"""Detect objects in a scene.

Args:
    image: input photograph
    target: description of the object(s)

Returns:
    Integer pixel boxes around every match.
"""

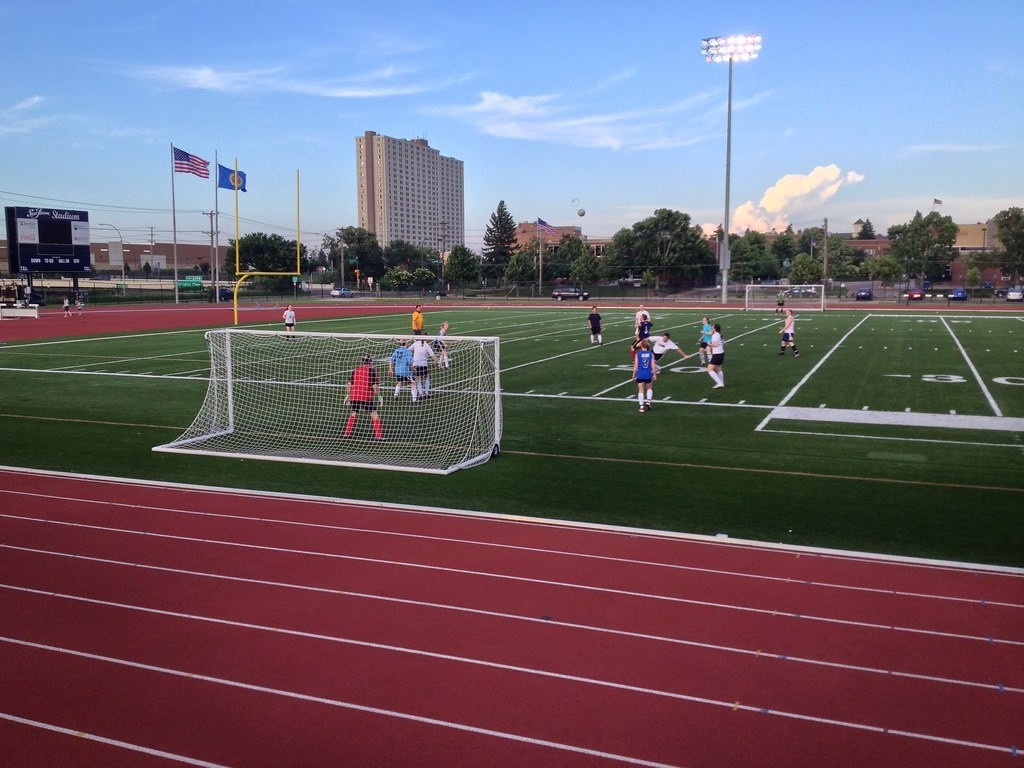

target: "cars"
[982,280,994,288]
[923,281,933,290]
[908,288,924,301]
[951,288,967,300]
[1007,289,1023,301]
[856,288,873,300]
[783,286,819,298]
[330,288,355,298]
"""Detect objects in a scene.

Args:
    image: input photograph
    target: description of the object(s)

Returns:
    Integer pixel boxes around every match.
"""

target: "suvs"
[210,288,233,301]
[552,288,590,301]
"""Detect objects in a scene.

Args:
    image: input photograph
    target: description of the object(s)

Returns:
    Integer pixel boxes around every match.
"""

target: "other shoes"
[424,393,435,399]
[341,437,353,442]
[411,399,419,404]
[438,365,445,370]
[418,394,426,399]
[443,366,453,370]
[639,406,646,413]
[375,437,387,443]
[393,396,399,401]
[774,313,783,316]
[712,384,725,388]
[794,353,800,358]
[700,362,708,366]
[646,401,651,410]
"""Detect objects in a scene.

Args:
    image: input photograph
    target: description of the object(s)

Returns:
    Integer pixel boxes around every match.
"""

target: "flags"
[537,218,557,236]
[812,240,817,247]
[174,146,210,179]
[218,164,246,193]
[935,199,942,204]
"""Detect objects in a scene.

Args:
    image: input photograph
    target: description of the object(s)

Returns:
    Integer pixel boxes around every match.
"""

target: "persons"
[282,304,297,343]
[389,338,420,404]
[63,295,72,317]
[411,305,424,342]
[776,289,785,313]
[707,323,726,388]
[628,304,689,375]
[408,331,438,400]
[375,279,380,298]
[778,309,800,358]
[588,306,603,345]
[631,340,657,413]
[75,299,82,315]
[698,316,713,366]
[343,354,383,440]
[434,320,451,369]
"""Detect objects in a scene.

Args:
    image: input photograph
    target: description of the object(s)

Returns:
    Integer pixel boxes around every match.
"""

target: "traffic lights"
[357,270,361,276]
[355,270,357,276]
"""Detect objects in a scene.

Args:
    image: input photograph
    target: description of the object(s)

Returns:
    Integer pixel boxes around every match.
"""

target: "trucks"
[995,284,1024,297]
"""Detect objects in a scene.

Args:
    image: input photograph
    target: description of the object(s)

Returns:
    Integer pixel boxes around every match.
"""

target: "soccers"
[578,208,585,217]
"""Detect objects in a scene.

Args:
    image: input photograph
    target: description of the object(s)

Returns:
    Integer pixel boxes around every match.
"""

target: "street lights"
[99,223,126,298]
[981,228,987,304]
[702,34,763,305]
[421,239,428,292]
